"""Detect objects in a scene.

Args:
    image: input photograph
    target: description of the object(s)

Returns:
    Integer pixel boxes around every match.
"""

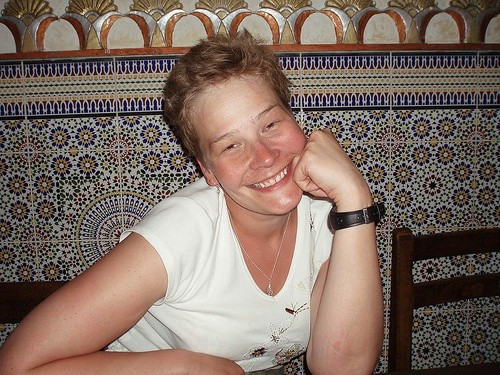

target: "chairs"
[386,226,500,375]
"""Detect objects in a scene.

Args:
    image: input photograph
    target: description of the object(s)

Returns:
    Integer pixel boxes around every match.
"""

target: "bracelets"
[329,201,387,230]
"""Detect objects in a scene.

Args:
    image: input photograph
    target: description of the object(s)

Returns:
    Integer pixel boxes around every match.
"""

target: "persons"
[0,29,385,375]
